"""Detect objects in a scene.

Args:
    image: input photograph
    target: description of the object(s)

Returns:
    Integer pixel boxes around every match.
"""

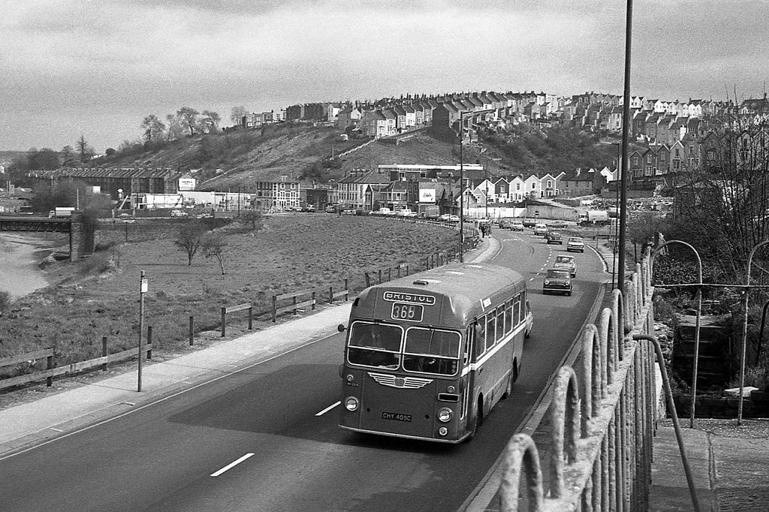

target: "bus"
[335,262,535,451]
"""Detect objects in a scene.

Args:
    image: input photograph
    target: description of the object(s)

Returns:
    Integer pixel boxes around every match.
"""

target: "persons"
[481,224,485,238]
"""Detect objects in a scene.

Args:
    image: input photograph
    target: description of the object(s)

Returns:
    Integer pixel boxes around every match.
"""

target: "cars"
[322,201,460,223]
[497,217,564,245]
[542,255,577,296]
[567,236,584,253]
